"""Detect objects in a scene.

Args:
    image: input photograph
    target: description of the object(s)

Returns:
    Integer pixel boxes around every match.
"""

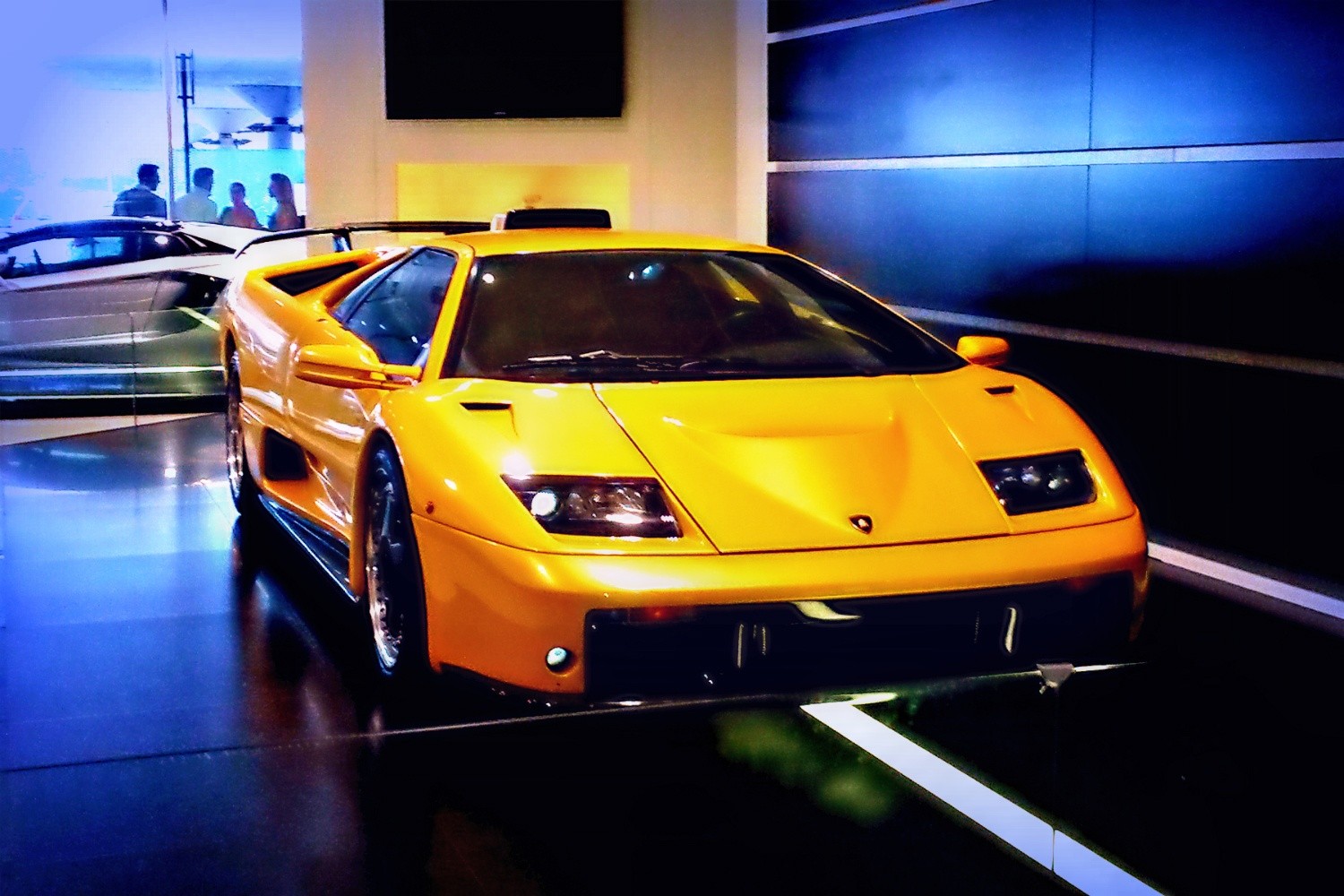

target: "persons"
[219,181,262,229]
[111,163,167,219]
[266,172,298,230]
[172,167,219,223]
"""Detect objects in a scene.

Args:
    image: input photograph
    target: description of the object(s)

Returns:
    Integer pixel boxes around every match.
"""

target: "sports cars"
[0,215,277,367]
[217,204,1151,706]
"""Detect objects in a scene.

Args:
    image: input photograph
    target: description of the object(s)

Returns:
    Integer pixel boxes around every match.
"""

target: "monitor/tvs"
[384,0,625,118]
